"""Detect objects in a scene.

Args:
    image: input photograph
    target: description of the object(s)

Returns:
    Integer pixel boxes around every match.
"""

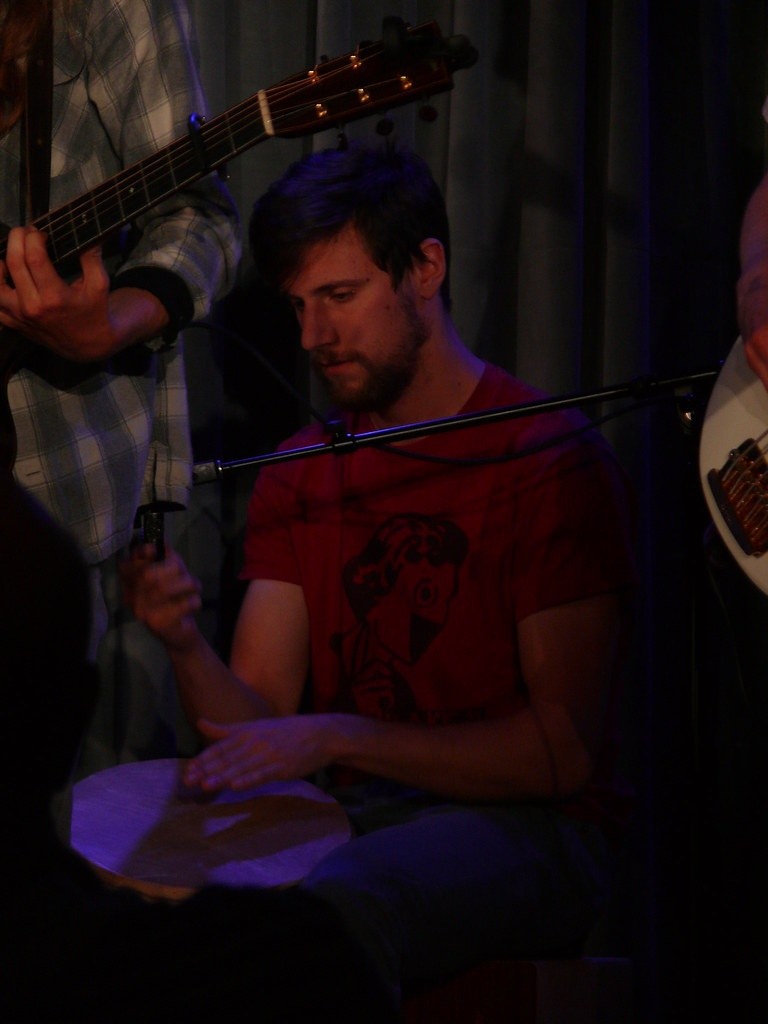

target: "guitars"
[0,17,479,305]
[700,334,768,596]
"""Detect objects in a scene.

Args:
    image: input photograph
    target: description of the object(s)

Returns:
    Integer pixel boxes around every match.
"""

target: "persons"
[68,149,619,1023]
[0,0,241,786]
[737,172,768,389]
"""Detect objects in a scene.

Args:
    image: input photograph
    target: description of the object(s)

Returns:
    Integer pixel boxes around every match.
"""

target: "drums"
[57,759,353,906]
